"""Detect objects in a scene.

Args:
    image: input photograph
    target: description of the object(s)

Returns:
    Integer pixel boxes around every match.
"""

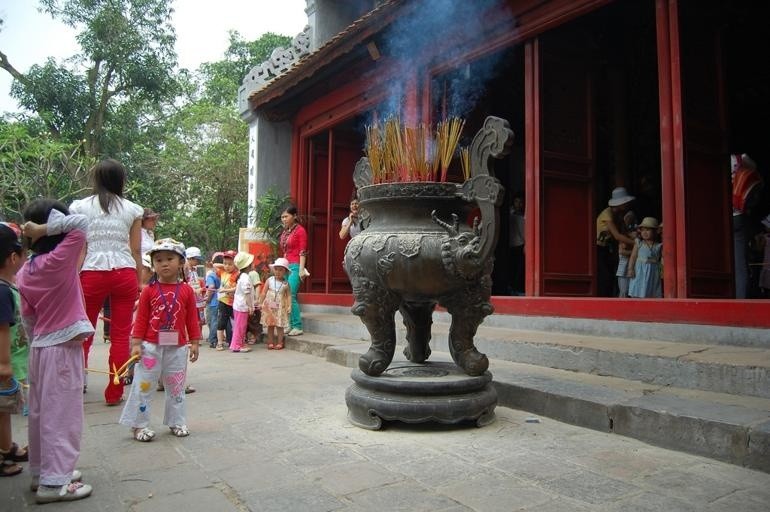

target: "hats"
[185,247,203,259]
[212,248,255,269]
[268,258,290,274]
[608,187,636,208]
[145,237,186,265]
[142,207,161,221]
[637,216,660,230]
[0,221,24,251]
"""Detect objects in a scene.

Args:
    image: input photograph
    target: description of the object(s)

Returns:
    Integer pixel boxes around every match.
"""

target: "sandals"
[0,441,29,477]
[266,342,285,350]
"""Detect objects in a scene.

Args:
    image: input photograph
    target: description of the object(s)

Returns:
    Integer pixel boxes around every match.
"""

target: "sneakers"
[156,383,196,394]
[124,375,134,385]
[30,469,93,504]
[210,338,256,352]
[284,326,304,336]
[133,428,156,442]
[168,423,190,438]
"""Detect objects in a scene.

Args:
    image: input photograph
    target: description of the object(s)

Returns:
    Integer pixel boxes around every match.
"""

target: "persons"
[492,194,525,296]
[731,153,770,299]
[14,198,92,503]
[68,159,145,406]
[597,187,663,298]
[339,193,361,239]
[0,222,31,476]
